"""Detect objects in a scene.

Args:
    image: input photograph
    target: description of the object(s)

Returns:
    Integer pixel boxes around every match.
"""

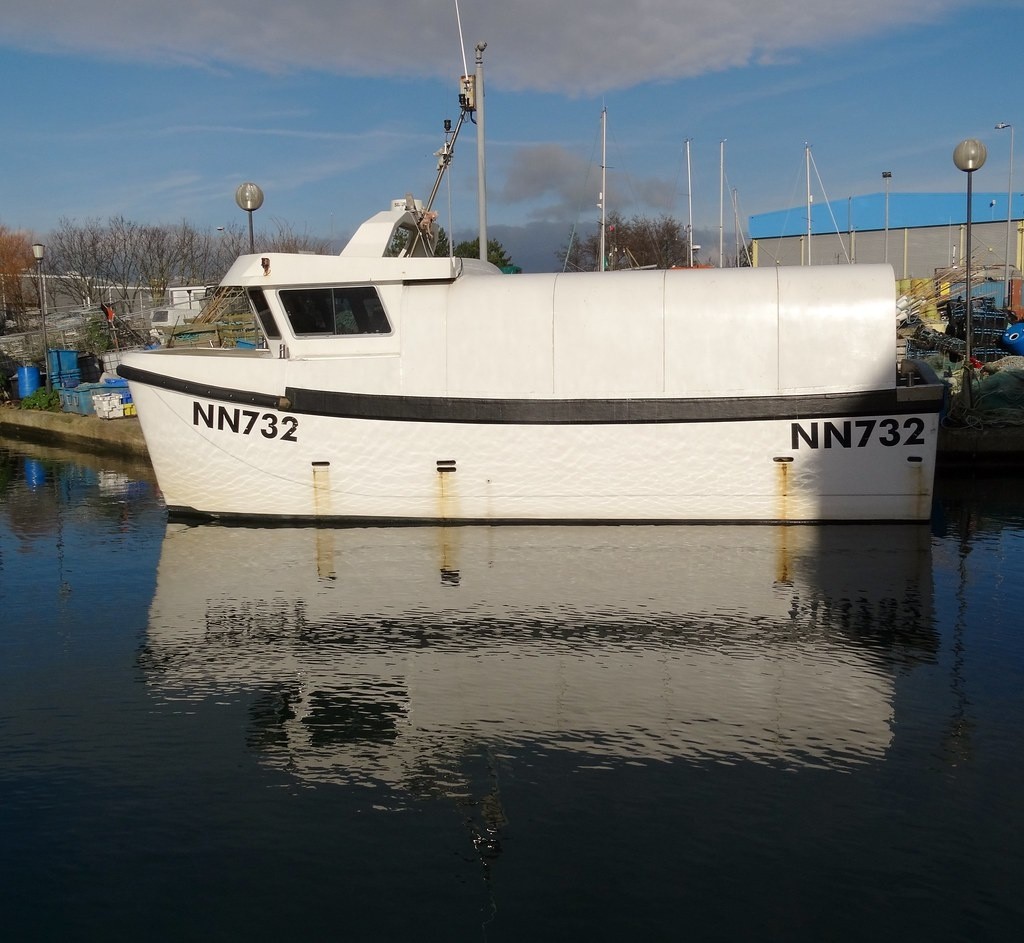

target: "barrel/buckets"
[1002,323,1024,357]
[18,366,41,399]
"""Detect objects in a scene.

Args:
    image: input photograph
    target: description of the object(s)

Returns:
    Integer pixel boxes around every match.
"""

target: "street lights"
[234,181,264,255]
[881,171,893,264]
[31,242,52,395]
[992,122,1015,309]
[951,138,988,411]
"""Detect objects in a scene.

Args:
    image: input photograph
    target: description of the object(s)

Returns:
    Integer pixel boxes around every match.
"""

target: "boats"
[115,1,946,526]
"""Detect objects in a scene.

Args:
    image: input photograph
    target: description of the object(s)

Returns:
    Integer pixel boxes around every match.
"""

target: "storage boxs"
[235,338,257,348]
[48,348,137,419]
